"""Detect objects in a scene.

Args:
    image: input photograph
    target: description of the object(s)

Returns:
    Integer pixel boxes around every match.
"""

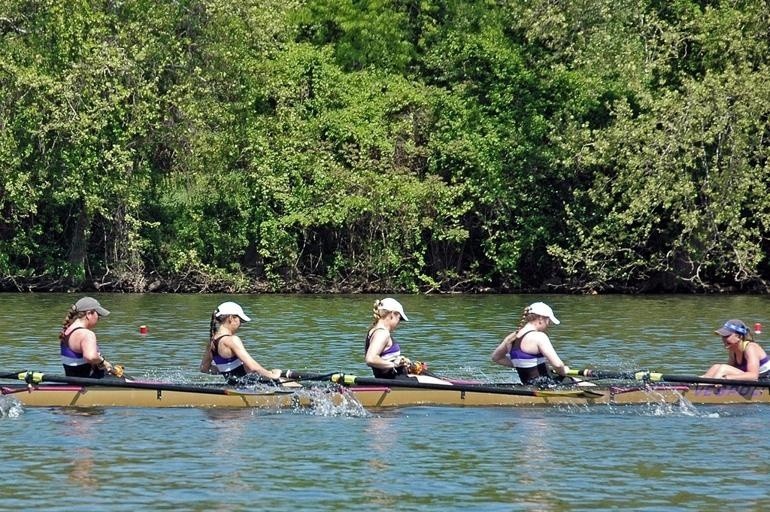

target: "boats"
[0,362,770,419]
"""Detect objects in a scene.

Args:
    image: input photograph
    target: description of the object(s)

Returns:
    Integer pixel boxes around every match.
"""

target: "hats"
[215,303,251,324]
[715,319,747,337]
[76,297,111,317]
[527,302,560,326]
[376,298,409,322]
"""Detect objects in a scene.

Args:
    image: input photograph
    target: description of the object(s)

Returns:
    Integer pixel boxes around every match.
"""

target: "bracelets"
[99,357,105,364]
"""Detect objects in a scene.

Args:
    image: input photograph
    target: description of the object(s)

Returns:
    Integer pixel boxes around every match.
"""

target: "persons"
[199,301,303,388]
[489,301,597,388]
[362,297,454,386]
[57,296,127,387]
[694,317,770,388]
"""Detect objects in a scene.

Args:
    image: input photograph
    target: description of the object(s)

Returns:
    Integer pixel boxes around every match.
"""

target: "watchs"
[722,375,728,380]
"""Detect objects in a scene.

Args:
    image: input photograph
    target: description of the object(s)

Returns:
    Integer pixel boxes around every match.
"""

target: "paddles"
[0,369,294,396]
[280,370,604,399]
